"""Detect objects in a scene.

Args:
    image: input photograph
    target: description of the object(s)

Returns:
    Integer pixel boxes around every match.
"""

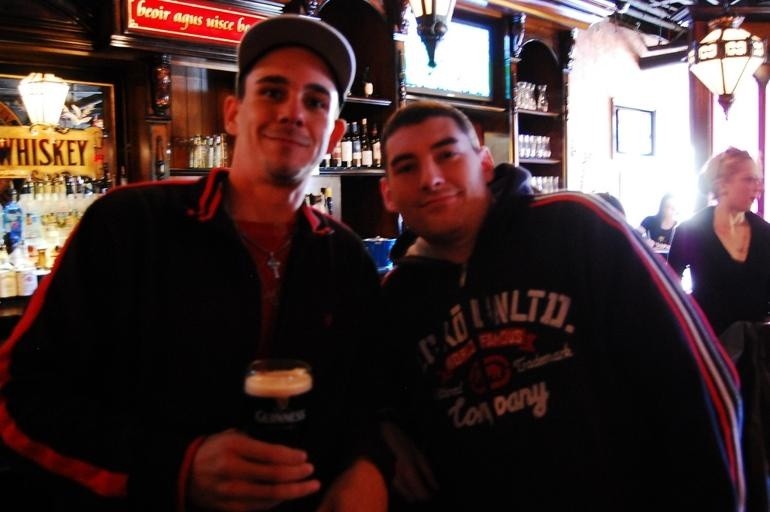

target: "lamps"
[687,0,764,120]
[17,72,70,135]
[409,0,456,67]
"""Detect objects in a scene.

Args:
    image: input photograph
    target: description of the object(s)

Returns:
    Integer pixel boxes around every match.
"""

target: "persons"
[665,147,770,512]
[588,191,625,218]
[0,15,392,512]
[374,100,747,512]
[637,194,684,247]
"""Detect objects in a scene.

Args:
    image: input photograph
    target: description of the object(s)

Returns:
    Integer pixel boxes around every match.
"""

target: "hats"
[237,15,357,104]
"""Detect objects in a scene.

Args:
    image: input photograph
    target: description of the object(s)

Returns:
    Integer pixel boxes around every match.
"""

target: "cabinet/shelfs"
[107,1,617,236]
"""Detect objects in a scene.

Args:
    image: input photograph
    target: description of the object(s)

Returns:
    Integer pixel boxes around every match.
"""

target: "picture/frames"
[610,97,659,160]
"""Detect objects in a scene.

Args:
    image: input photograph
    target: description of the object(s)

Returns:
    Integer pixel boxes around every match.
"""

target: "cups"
[244,365,314,454]
[361,237,397,274]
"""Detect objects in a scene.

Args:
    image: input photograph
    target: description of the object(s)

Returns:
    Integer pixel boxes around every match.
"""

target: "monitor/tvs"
[405,14,496,104]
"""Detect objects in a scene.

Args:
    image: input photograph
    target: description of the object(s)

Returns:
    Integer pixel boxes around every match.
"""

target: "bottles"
[155,134,167,179]
[304,118,387,217]
[362,59,378,101]
[516,80,560,198]
[188,133,233,169]
[0,166,128,313]
